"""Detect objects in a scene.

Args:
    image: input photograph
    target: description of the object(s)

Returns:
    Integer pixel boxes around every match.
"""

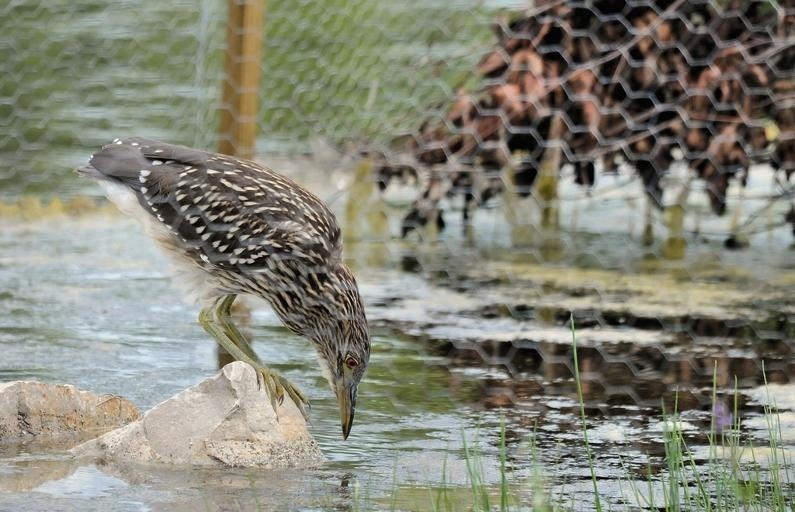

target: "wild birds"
[74,136,370,441]
